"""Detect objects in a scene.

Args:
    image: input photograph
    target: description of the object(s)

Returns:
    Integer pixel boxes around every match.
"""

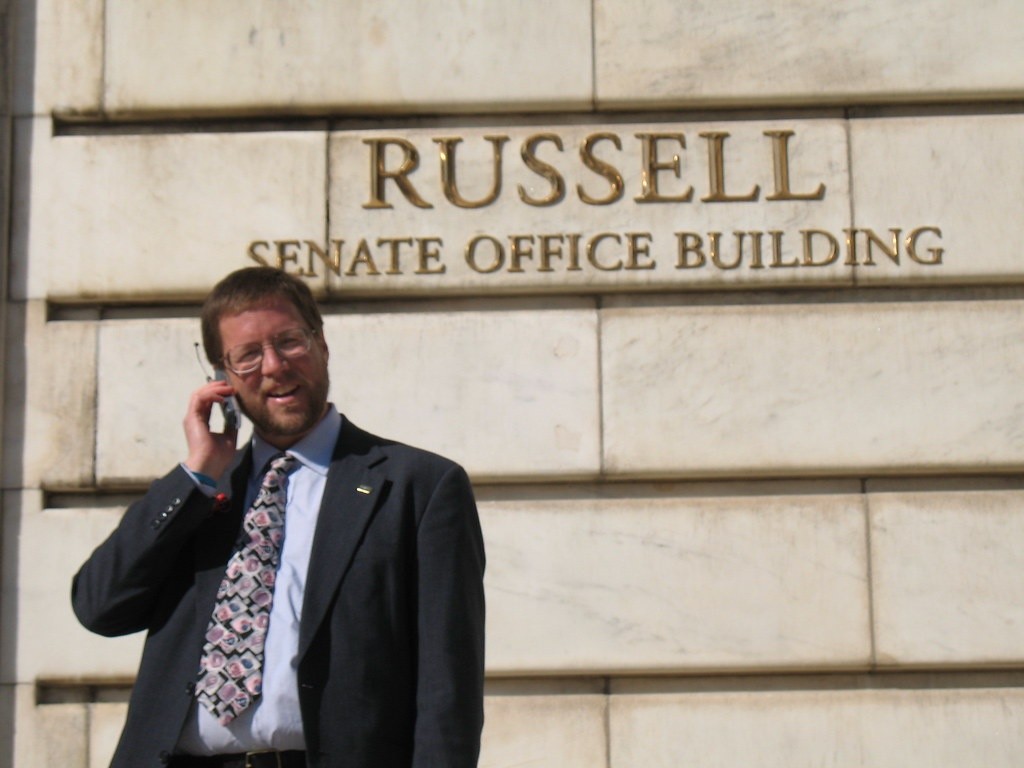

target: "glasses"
[219,327,318,374]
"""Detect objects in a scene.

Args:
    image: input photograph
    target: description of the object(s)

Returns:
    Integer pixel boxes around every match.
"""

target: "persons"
[70,265,486,768]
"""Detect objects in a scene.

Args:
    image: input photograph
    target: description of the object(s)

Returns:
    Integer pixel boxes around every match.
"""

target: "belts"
[169,749,309,768]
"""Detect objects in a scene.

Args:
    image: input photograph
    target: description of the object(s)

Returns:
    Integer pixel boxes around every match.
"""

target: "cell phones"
[194,342,242,431]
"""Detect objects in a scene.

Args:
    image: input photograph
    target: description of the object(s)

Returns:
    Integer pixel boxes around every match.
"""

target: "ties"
[194,454,296,727]
